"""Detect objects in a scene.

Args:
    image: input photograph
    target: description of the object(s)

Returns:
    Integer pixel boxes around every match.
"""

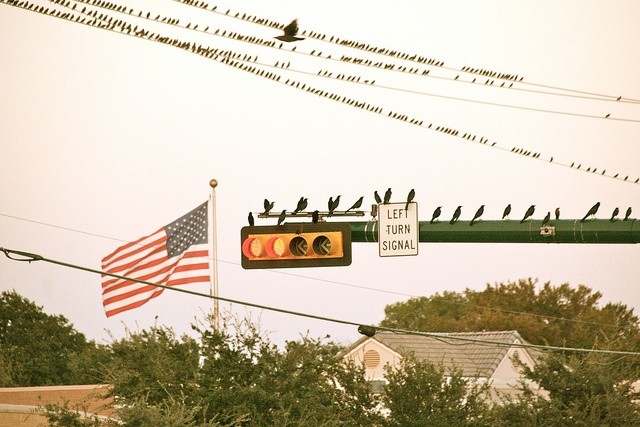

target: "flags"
[100,200,210,317]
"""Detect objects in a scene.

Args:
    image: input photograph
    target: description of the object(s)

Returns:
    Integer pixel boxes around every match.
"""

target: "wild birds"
[427,205,443,225]
[580,200,601,222]
[449,205,463,227]
[403,188,416,211]
[522,203,537,224]
[245,212,260,227]
[259,197,275,216]
[383,187,392,206]
[326,194,343,213]
[623,205,633,225]
[276,209,288,229]
[293,195,309,214]
[0,0,639,187]
[540,212,552,230]
[371,189,384,209]
[499,203,513,221]
[556,206,561,220]
[345,196,364,214]
[610,207,621,224]
[469,204,487,228]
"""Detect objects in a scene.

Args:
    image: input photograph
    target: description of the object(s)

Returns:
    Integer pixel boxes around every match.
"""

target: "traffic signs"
[378,202,418,256]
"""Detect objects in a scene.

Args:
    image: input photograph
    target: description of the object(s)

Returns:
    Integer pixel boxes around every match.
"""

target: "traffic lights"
[240,227,352,270]
